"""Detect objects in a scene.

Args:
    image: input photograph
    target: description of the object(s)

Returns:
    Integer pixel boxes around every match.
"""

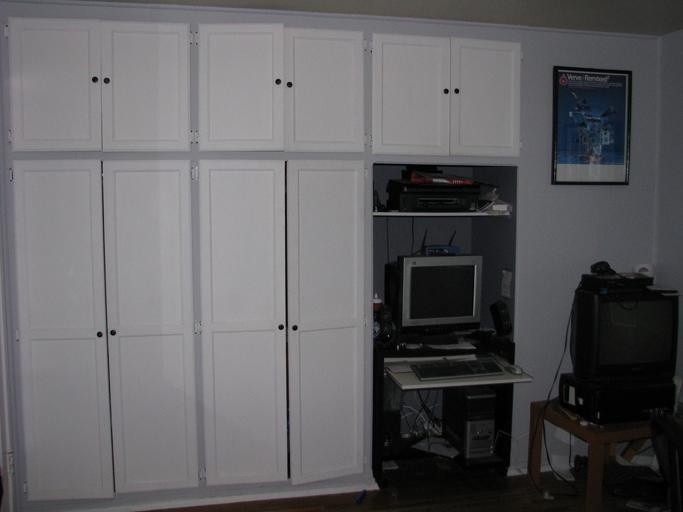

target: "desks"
[528,401,683,512]
[384,342,533,466]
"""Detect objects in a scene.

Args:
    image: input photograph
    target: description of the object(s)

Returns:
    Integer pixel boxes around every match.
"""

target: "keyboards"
[412,358,504,381]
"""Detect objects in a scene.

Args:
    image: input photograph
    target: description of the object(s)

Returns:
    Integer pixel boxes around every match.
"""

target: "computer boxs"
[443,387,497,459]
[559,373,674,426]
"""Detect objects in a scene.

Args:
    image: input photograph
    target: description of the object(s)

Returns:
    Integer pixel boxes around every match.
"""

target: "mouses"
[506,365,523,375]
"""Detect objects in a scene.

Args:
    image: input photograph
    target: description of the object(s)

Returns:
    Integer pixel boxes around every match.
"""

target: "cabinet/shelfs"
[367,15,522,157]
[195,156,380,508]
[0,1,195,156]
[1,156,195,511]
[195,6,367,157]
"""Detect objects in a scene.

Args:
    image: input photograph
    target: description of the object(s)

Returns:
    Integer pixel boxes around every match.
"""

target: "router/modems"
[420,228,458,255]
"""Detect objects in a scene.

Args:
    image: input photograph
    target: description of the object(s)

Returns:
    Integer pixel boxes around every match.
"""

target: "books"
[411,170,474,185]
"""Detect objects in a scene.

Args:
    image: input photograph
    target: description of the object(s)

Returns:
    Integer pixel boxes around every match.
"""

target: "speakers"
[492,302,512,335]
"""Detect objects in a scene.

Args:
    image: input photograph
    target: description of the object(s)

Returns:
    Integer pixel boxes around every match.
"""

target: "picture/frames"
[552,66,632,185]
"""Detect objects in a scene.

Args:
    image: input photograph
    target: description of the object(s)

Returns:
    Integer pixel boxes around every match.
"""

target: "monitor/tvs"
[384,256,484,334]
[569,289,677,381]
